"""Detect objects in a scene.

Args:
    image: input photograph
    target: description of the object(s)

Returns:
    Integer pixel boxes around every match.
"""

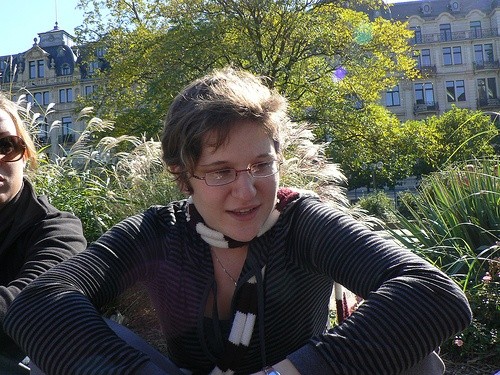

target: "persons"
[1,70,473,375]
[0,96,83,375]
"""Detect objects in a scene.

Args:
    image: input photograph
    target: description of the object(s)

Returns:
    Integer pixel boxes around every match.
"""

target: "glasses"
[0,135,29,162]
[182,148,283,186]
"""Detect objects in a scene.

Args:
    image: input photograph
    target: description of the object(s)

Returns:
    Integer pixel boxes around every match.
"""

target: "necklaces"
[206,242,237,286]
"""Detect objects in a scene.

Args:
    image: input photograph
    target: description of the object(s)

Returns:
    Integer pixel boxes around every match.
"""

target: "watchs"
[263,366,280,375]
